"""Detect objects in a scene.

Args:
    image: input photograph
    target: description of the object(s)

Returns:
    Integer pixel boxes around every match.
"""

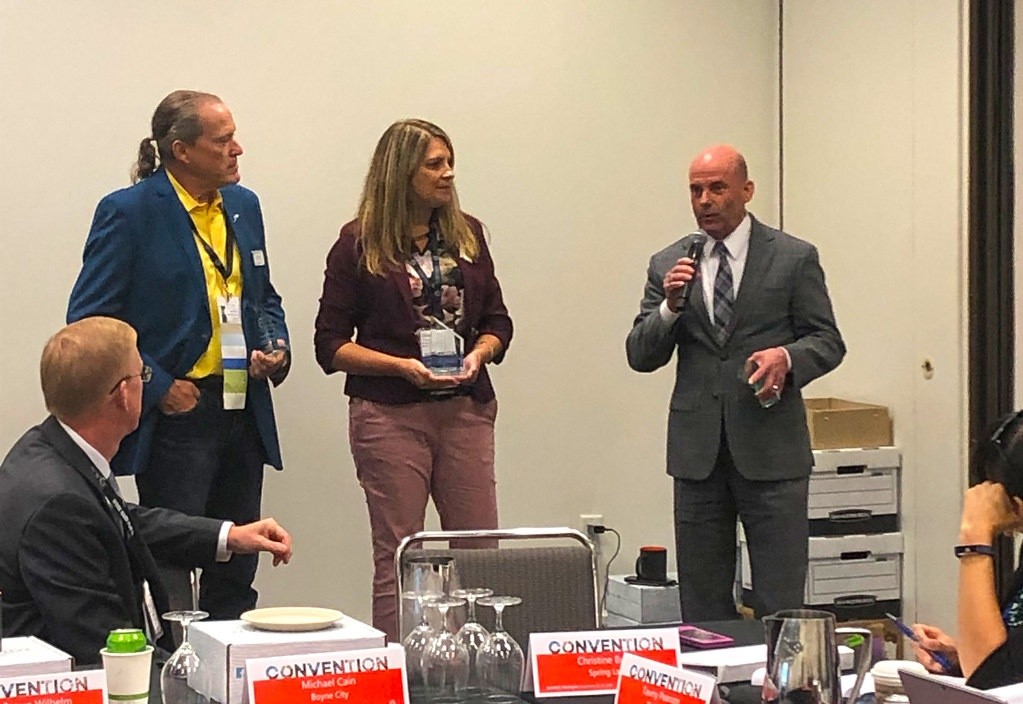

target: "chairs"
[393,528,598,654]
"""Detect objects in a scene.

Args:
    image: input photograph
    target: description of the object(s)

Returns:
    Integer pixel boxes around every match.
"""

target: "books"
[1,635,78,680]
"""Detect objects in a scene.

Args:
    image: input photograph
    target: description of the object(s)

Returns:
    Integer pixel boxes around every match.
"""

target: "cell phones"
[678,626,733,650]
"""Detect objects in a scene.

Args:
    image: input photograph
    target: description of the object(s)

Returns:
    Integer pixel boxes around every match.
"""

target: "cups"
[636,547,667,581]
[870,661,928,704]
[100,646,154,704]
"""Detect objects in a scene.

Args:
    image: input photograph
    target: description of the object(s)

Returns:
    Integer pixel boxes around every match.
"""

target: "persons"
[908,411,1023,691]
[625,146,846,626]
[0,315,294,667]
[315,117,515,644]
[65,90,291,646]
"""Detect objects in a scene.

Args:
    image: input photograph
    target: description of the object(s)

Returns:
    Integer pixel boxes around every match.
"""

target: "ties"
[713,242,735,347]
[107,471,126,537]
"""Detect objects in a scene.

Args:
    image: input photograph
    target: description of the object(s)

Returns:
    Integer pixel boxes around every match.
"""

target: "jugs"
[762,609,872,704]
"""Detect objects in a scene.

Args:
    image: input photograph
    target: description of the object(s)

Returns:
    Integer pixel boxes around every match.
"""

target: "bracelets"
[475,342,494,364]
[954,544,996,559]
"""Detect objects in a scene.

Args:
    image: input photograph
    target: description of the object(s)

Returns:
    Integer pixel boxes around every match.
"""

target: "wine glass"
[402,591,446,696]
[449,588,494,696]
[421,597,470,704]
[160,611,211,704]
[475,596,525,703]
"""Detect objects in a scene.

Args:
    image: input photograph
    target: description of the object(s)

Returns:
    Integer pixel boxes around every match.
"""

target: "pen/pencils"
[885,612,953,673]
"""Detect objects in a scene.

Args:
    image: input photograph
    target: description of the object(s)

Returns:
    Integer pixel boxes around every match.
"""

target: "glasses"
[990,407,1023,494]
[109,365,154,396]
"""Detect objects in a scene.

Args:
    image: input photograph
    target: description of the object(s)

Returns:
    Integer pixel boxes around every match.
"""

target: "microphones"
[675,231,708,311]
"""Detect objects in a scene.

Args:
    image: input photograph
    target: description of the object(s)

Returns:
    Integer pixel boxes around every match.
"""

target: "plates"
[240,607,343,631]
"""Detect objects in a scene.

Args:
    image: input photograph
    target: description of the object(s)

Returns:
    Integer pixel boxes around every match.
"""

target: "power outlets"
[580,514,602,554]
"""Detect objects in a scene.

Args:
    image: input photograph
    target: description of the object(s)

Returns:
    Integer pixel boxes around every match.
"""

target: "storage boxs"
[734,398,903,661]
[0,636,78,680]
[187,615,389,704]
[603,572,682,628]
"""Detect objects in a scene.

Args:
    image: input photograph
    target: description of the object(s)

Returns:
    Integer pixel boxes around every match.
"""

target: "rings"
[773,384,779,390]
